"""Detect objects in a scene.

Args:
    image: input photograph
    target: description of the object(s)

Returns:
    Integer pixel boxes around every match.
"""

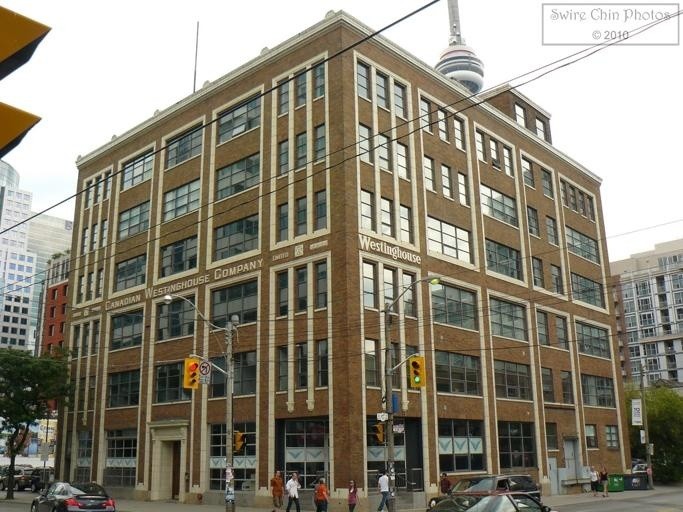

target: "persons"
[599,466,610,498]
[376,469,390,512]
[590,465,601,497]
[439,471,451,495]
[285,472,302,512]
[347,479,359,512]
[270,469,285,511]
[313,477,329,512]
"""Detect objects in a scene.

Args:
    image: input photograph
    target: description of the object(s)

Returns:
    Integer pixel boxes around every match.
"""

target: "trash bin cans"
[590,472,648,491]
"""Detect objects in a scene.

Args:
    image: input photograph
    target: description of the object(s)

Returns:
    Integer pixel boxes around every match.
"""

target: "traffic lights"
[234,432,248,455]
[371,424,383,445]
[409,356,426,387]
[182,357,199,390]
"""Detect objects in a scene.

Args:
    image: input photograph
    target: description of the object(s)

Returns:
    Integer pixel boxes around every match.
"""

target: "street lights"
[164,294,234,512]
[381,275,441,512]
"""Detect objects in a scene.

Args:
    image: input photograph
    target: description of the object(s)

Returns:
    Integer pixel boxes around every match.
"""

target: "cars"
[426,474,550,512]
[0,465,55,492]
[30,480,115,512]
